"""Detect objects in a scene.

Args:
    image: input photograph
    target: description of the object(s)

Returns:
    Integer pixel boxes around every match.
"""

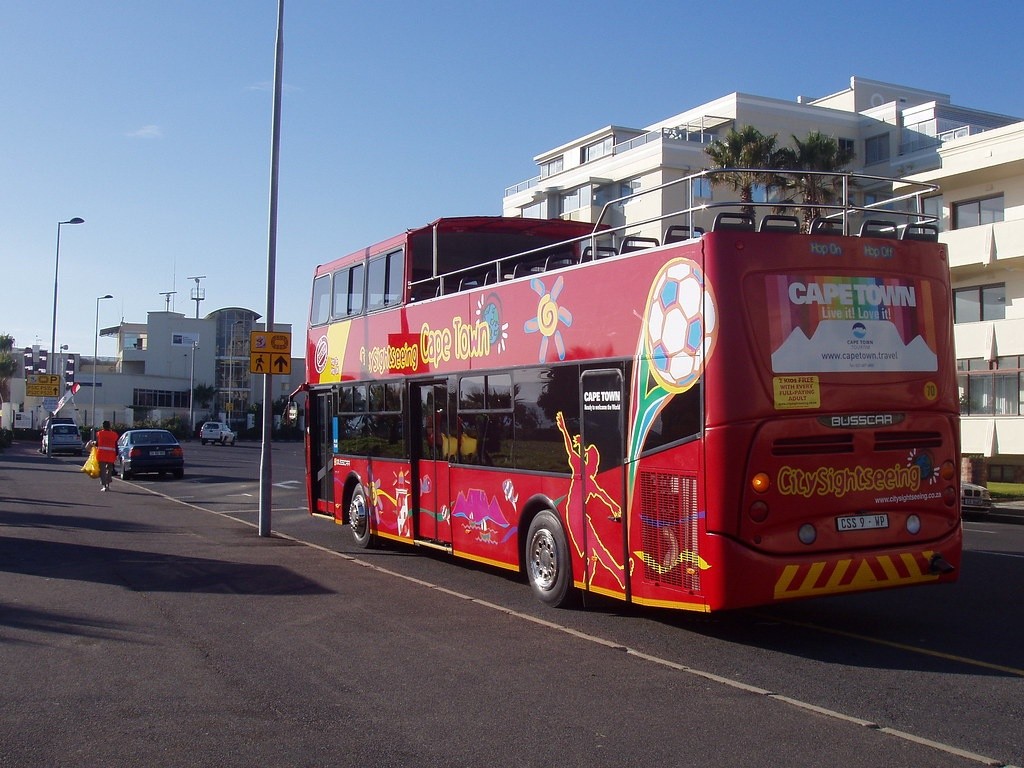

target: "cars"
[112,429,185,479]
[961,479,994,511]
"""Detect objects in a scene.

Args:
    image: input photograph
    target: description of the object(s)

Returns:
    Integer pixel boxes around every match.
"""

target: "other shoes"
[106,485,109,491]
[100,488,106,493]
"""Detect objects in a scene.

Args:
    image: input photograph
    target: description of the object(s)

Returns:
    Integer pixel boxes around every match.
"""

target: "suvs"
[40,416,75,437]
[40,423,84,456]
[199,422,235,447]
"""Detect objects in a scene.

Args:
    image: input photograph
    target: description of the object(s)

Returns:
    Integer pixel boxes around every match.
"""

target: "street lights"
[59,344,68,374]
[48,217,85,415]
[228,320,243,430]
[92,294,113,440]
[184,340,200,441]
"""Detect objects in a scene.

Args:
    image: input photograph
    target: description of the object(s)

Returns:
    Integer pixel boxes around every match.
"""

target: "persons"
[93,420,119,493]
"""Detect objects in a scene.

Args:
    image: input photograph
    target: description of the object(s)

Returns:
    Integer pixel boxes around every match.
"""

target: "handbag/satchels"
[81,446,101,479]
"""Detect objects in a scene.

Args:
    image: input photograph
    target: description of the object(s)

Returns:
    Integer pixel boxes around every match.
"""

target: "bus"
[286,170,964,614]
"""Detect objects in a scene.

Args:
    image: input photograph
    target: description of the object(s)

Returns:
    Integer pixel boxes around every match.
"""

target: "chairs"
[581,246,619,264]
[513,261,545,279]
[809,217,850,236]
[619,236,660,255]
[54,428,60,433]
[435,284,456,297]
[859,220,899,239]
[662,225,706,245]
[901,223,939,242]
[758,214,800,233]
[458,277,484,293]
[712,212,755,232]
[484,270,496,286]
[545,256,580,272]
